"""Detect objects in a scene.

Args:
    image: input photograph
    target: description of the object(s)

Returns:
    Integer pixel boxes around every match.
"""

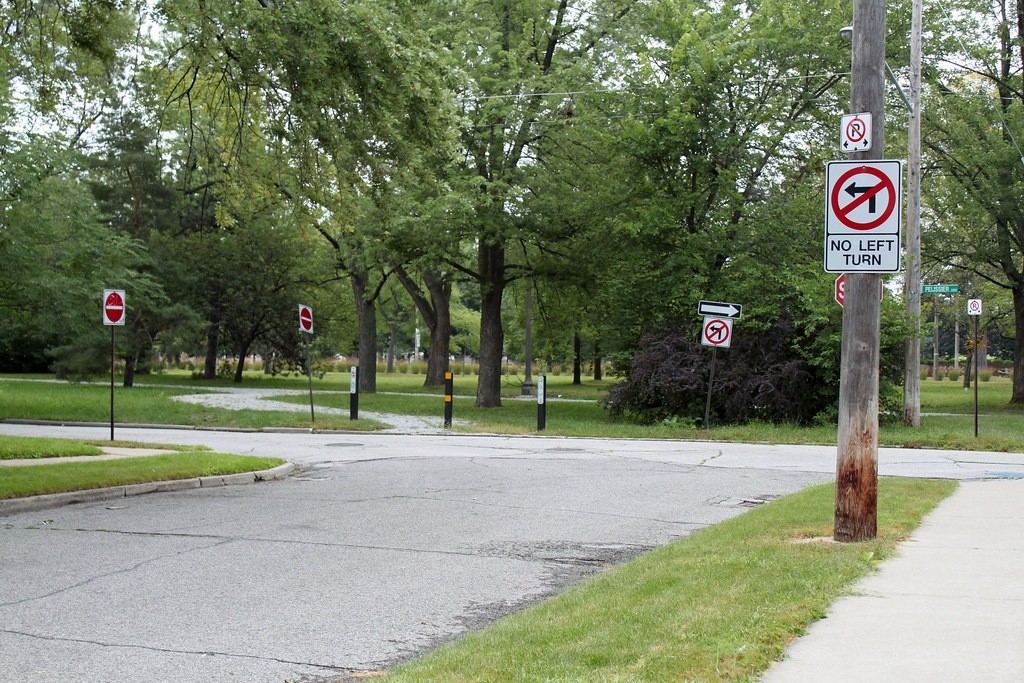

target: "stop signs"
[835,273,883,308]
[103,289,125,325]
[299,304,313,334]
